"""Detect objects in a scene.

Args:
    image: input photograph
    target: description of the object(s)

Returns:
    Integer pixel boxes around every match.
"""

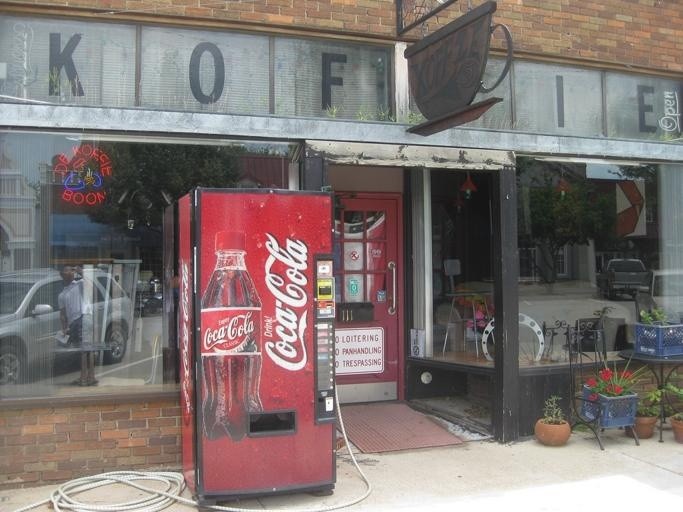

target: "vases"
[580,384,638,431]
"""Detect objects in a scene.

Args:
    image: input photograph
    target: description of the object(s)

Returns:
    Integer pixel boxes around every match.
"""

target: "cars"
[635,268,682,323]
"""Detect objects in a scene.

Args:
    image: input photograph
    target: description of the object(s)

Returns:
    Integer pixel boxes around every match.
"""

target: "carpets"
[333,400,467,455]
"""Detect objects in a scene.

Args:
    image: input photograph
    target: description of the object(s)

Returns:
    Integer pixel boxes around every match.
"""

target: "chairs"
[95,330,160,389]
[438,255,496,360]
[537,316,581,364]
[561,314,644,451]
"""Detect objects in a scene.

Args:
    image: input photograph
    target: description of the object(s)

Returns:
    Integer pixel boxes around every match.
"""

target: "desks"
[47,340,112,386]
[613,351,683,426]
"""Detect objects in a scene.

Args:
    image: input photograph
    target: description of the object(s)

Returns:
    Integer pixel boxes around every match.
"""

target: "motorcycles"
[138,276,162,316]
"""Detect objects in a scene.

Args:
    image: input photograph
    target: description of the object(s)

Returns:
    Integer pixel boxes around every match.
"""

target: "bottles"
[198,229,265,443]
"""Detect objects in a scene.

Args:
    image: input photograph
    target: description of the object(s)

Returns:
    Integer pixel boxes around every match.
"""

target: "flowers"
[463,308,487,330]
[577,342,653,406]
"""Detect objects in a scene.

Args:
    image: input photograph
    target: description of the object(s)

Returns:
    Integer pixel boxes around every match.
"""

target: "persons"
[58,264,97,380]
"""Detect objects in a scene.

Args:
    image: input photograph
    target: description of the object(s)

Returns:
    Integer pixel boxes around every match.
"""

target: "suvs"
[0,267,132,384]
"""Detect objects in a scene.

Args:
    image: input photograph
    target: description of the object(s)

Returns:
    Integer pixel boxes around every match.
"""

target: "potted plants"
[661,377,682,446]
[529,388,573,448]
[624,384,666,445]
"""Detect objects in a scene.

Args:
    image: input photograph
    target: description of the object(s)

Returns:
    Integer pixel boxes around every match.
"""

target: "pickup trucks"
[596,258,649,298]
